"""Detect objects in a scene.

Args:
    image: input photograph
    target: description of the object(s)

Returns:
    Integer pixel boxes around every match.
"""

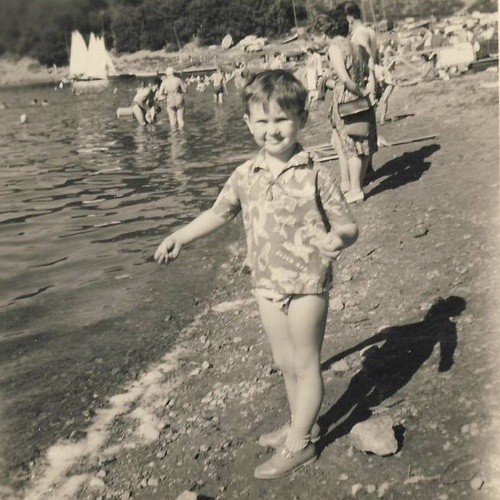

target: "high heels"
[344,191,365,204]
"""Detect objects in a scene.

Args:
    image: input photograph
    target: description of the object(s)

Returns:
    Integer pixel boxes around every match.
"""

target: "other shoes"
[254,439,318,480]
[258,418,322,447]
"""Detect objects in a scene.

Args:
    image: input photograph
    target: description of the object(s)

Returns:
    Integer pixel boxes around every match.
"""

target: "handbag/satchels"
[338,99,371,118]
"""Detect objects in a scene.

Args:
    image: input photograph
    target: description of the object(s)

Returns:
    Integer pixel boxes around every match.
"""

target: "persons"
[131,77,161,127]
[145,102,161,124]
[159,67,186,127]
[373,63,396,126]
[136,47,327,105]
[324,8,379,203]
[373,16,499,87]
[153,70,358,480]
[324,0,376,192]
